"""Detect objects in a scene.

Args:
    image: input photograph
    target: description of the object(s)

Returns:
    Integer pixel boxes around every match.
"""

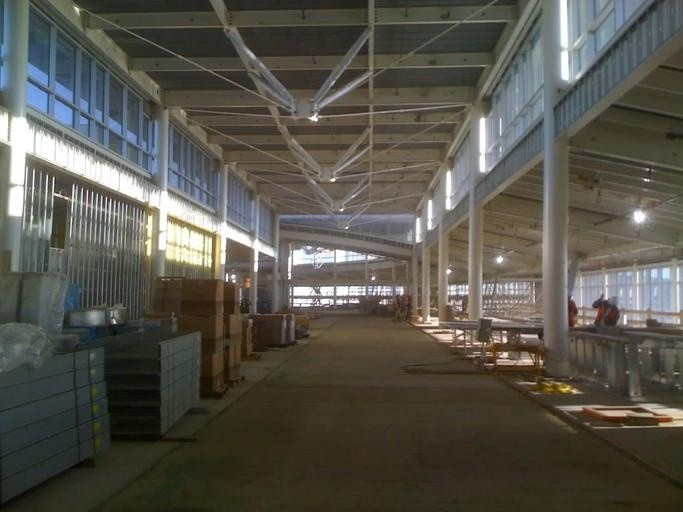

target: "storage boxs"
[151,277,255,398]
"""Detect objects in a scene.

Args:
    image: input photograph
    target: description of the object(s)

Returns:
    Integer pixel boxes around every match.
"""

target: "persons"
[592,292,620,327]
[394,291,411,322]
[568,294,578,327]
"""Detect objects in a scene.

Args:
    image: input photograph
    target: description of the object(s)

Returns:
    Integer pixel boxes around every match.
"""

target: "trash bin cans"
[439,305,455,328]
